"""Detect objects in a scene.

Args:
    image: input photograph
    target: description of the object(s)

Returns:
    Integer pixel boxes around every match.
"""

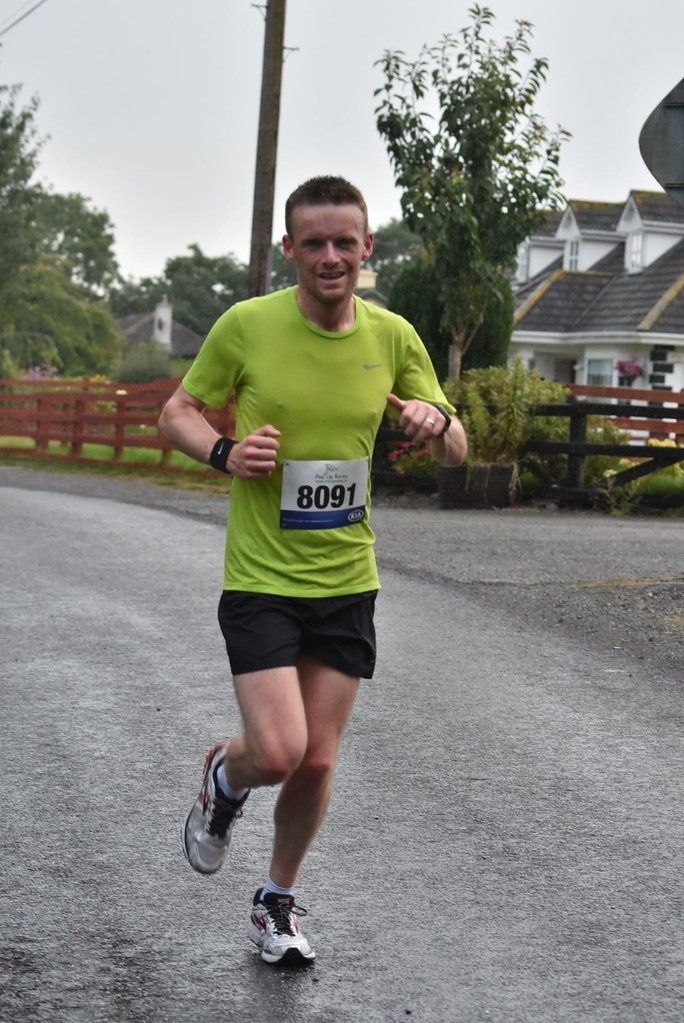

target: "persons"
[158,176,469,964]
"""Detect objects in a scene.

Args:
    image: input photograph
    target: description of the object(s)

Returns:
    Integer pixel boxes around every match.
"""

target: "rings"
[426,418,434,425]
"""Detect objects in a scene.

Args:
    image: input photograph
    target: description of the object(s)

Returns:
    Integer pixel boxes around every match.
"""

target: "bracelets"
[210,437,240,474]
[434,404,451,438]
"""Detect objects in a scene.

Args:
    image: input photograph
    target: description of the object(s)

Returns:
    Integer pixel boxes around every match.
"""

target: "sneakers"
[179,742,252,876]
[244,886,318,966]
